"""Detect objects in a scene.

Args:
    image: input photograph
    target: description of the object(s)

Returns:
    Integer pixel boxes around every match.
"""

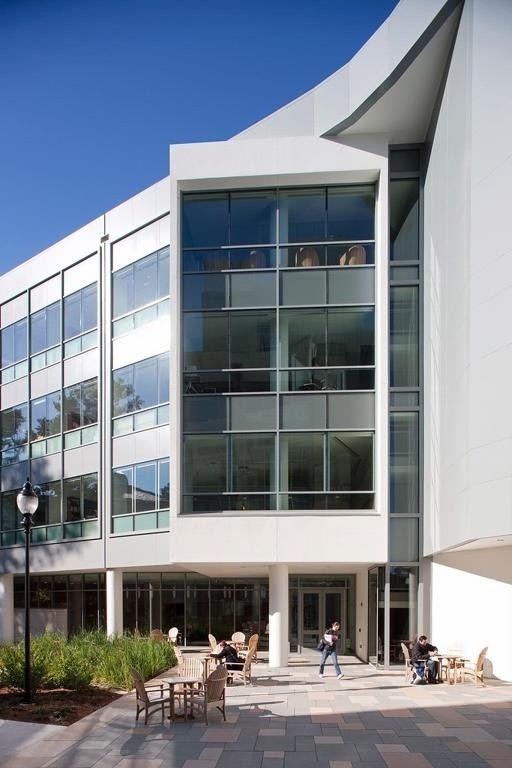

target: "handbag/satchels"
[317,642,323,649]
[236,657,245,669]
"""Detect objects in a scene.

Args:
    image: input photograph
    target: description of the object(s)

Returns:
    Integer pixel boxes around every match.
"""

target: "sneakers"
[319,673,323,677]
[337,673,344,679]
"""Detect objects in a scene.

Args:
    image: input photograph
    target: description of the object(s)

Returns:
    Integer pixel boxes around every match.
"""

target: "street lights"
[14,479,40,701]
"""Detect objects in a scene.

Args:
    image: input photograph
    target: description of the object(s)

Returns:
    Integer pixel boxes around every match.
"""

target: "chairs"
[129,627,259,725]
[378,638,488,685]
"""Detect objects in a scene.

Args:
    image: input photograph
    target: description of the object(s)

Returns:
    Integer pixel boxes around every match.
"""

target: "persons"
[412,636,438,684]
[207,641,240,682]
[318,621,344,680]
